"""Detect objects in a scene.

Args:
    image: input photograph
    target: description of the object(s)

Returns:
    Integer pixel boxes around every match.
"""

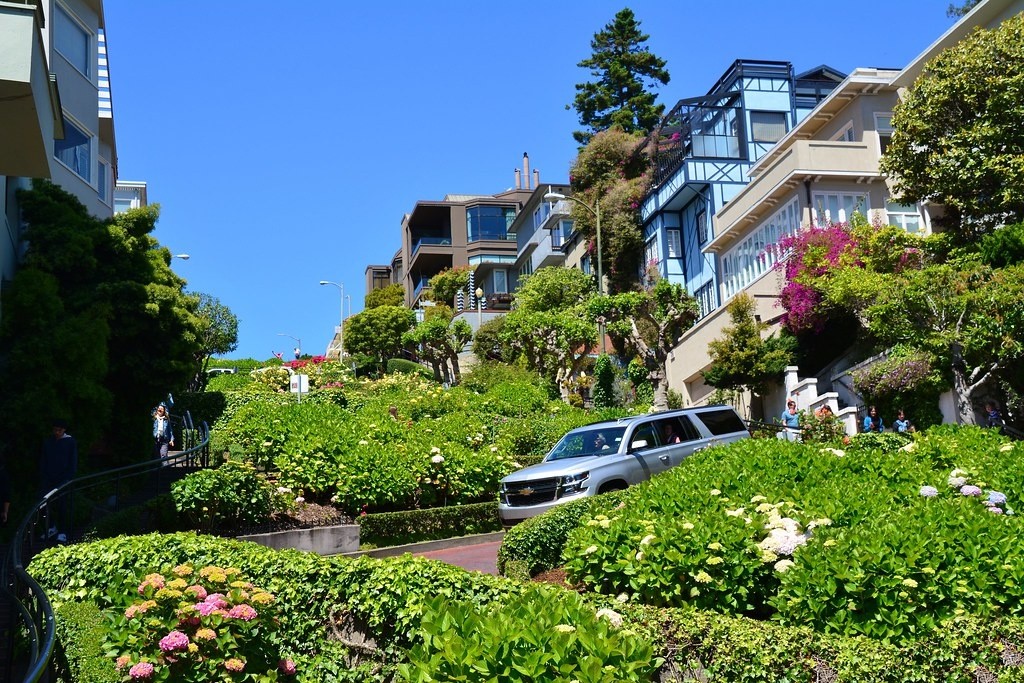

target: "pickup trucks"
[205,365,236,377]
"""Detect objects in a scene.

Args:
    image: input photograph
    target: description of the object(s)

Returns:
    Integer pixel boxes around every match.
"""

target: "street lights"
[278,334,301,405]
[345,295,351,319]
[544,192,605,355]
[320,280,344,363]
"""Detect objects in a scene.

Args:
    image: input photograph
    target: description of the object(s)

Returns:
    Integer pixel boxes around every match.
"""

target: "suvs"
[498,404,755,530]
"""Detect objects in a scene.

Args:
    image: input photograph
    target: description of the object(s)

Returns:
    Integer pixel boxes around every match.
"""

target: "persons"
[781,401,803,443]
[864,405,883,433]
[663,423,680,444]
[388,406,398,421]
[591,433,610,450]
[0,452,11,528]
[893,409,916,432]
[39,419,77,542]
[579,366,586,383]
[984,401,1006,436]
[294,347,300,359]
[151,384,174,467]
[277,354,282,360]
